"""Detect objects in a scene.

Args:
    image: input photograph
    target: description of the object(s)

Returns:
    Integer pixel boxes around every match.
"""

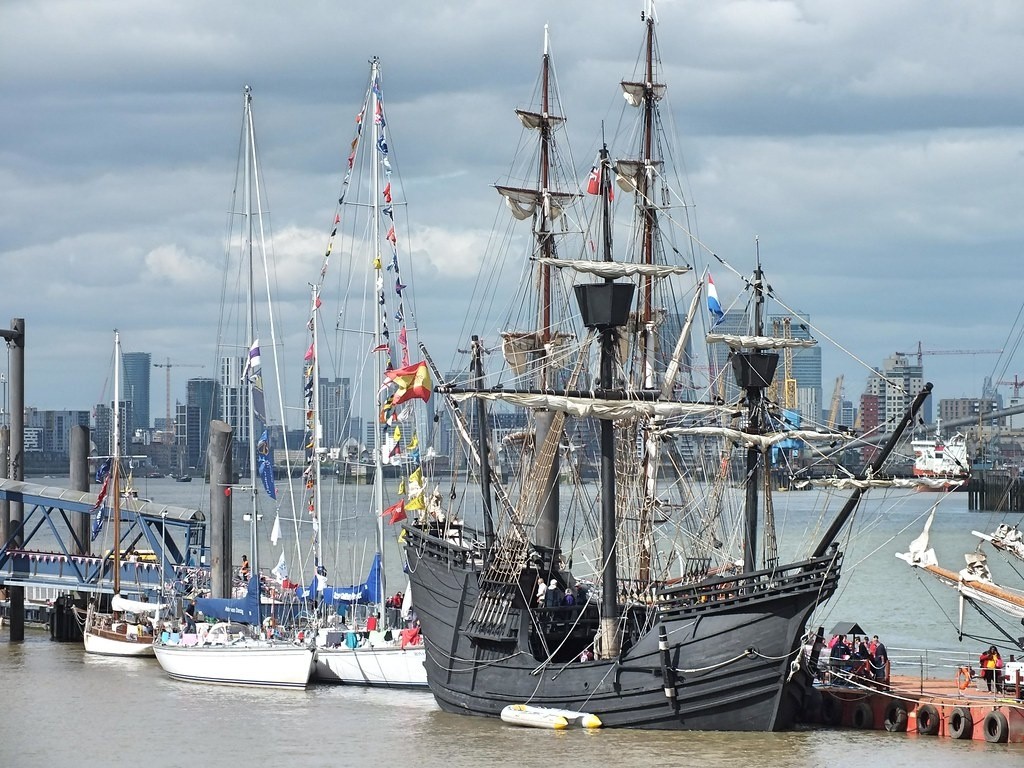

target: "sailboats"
[379,1,936,735]
[272,56,429,687]
[83,329,156,659]
[149,85,317,692]
[893,302,1024,690]
[176,449,192,482]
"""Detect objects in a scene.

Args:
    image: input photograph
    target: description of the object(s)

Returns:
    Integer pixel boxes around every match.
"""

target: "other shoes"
[988,691,991,694]
[997,691,1001,695]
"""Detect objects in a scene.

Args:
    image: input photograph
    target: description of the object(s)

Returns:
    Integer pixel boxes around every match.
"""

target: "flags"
[706,269,725,328]
[307,68,446,549]
[241,340,325,603]
[587,163,613,202]
[90,458,111,542]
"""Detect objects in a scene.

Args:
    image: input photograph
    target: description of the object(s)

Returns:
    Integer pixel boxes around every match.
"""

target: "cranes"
[896,341,1004,367]
[154,357,207,431]
[997,373,1024,397]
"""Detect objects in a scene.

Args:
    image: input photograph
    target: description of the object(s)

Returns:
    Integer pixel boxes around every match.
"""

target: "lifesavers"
[137,624,143,636]
[983,711,1008,743]
[955,668,970,689]
[883,700,907,732]
[947,707,973,739]
[822,696,842,725]
[917,704,940,735]
[851,702,874,729]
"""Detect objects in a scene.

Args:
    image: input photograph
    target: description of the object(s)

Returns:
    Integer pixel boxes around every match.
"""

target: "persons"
[127,545,140,563]
[535,577,586,630]
[809,634,889,686]
[185,599,197,634]
[138,619,155,636]
[385,592,405,610]
[980,646,1004,694]
[241,555,251,580]
[580,643,593,662]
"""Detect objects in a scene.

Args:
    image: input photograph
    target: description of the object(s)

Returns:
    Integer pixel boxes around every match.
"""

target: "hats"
[574,582,581,587]
[550,579,557,585]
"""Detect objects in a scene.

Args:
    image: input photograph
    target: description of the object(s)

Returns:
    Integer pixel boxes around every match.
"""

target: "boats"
[911,417,968,492]
[170,474,176,479]
[338,436,376,484]
[615,495,673,523]
